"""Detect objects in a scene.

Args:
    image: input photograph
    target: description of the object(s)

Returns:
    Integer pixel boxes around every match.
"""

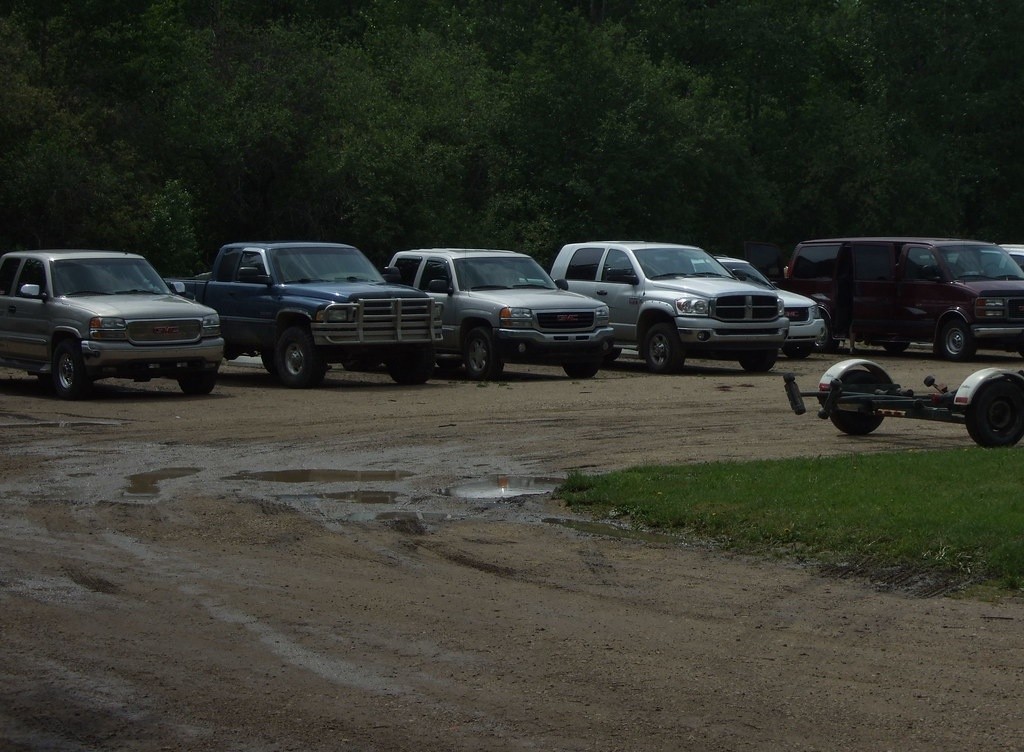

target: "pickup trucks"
[518,240,791,375]
[380,248,614,381]
[0,249,223,401]
[163,241,442,389]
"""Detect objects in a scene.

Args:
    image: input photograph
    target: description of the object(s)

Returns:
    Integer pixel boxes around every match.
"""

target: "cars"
[616,255,826,358]
[920,244,1024,278]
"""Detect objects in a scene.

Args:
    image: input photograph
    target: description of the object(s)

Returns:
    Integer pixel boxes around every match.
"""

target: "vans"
[783,236,1024,363]
